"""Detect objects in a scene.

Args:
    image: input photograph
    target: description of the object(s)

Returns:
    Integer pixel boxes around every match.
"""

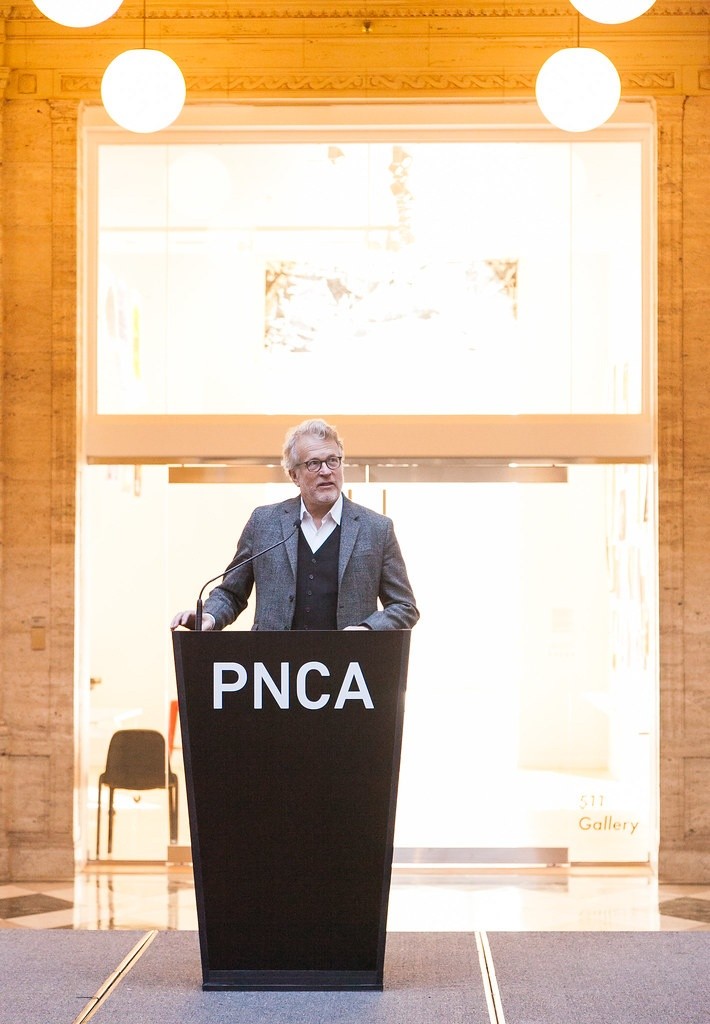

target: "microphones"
[195,518,301,631]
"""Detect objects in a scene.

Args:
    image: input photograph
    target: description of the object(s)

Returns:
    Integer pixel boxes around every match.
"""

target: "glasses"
[293,454,342,472]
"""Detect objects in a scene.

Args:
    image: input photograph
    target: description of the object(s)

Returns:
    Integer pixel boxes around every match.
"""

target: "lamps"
[535,11,621,133]
[570,0,656,25]
[33,0,123,28]
[101,0,186,133]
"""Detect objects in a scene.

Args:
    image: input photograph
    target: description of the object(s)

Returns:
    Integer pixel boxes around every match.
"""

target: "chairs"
[96,729,179,862]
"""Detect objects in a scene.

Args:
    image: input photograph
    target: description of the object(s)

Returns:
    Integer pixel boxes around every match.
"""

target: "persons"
[170,418,420,631]
